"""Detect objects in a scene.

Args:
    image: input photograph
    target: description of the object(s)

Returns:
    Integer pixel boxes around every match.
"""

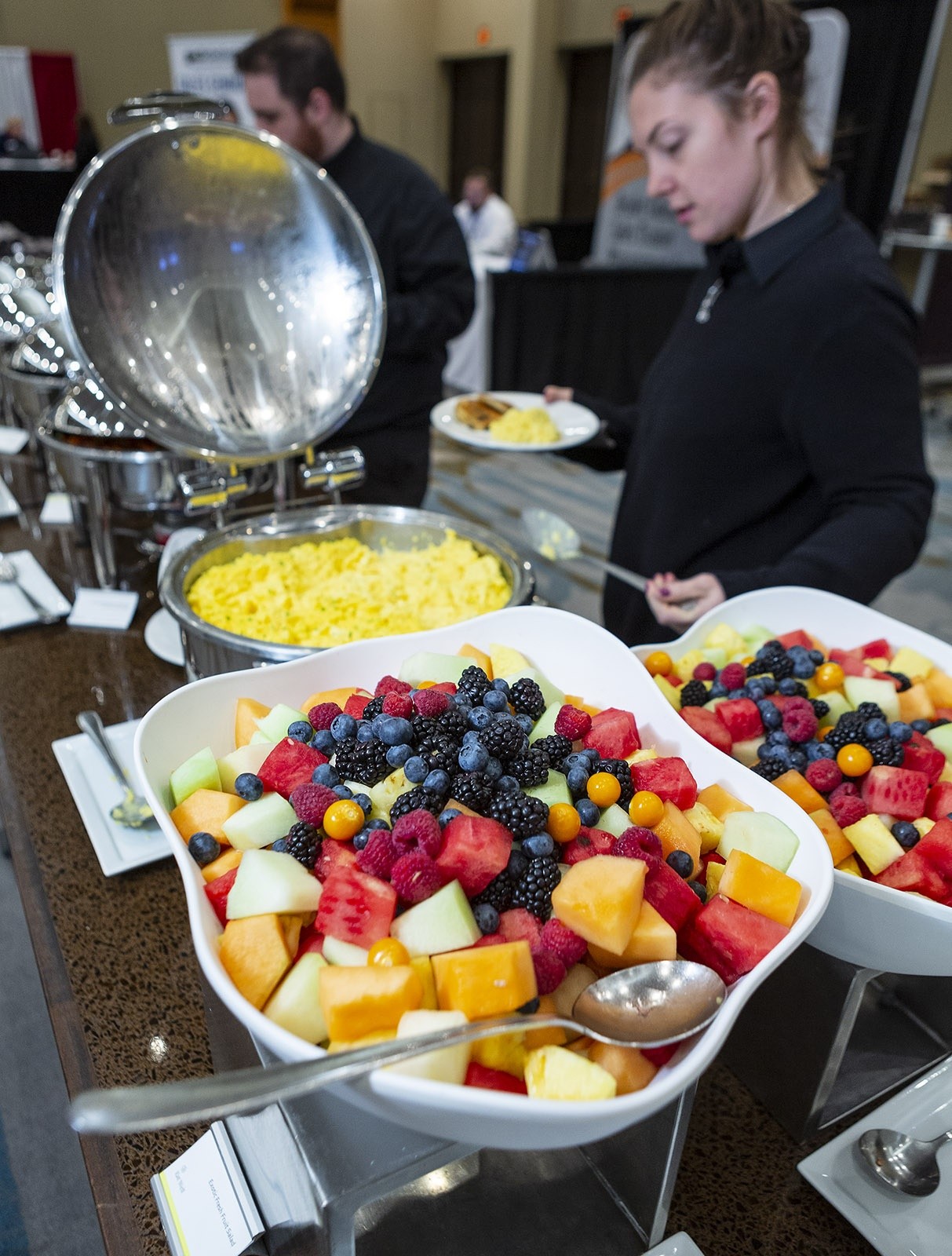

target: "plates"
[0,549,73,631]
[796,1055,951,1256]
[431,390,598,448]
[144,606,186,669]
[52,713,177,878]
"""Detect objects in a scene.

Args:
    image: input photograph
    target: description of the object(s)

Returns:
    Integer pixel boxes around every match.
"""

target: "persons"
[234,25,477,508]
[454,166,519,256]
[0,114,98,174]
[546,1,938,647]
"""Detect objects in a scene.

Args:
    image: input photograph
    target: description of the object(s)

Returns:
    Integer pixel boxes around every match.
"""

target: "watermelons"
[204,628,952,1088]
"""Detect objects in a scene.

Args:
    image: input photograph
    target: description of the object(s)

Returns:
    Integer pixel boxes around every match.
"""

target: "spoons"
[74,711,157,827]
[520,507,658,594]
[0,551,60,624]
[859,1126,952,1198]
[62,961,727,1147]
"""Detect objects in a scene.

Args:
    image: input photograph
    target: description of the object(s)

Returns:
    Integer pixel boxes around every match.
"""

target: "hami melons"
[655,624,952,877]
[171,644,805,1097]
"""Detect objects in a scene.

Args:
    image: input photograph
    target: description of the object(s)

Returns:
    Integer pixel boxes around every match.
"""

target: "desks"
[0,505,881,1255]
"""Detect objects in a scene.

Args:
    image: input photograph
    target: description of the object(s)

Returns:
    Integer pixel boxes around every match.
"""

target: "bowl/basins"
[133,606,835,1147]
[627,584,952,982]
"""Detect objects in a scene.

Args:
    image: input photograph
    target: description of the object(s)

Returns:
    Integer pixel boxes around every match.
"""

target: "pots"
[0,93,537,684]
